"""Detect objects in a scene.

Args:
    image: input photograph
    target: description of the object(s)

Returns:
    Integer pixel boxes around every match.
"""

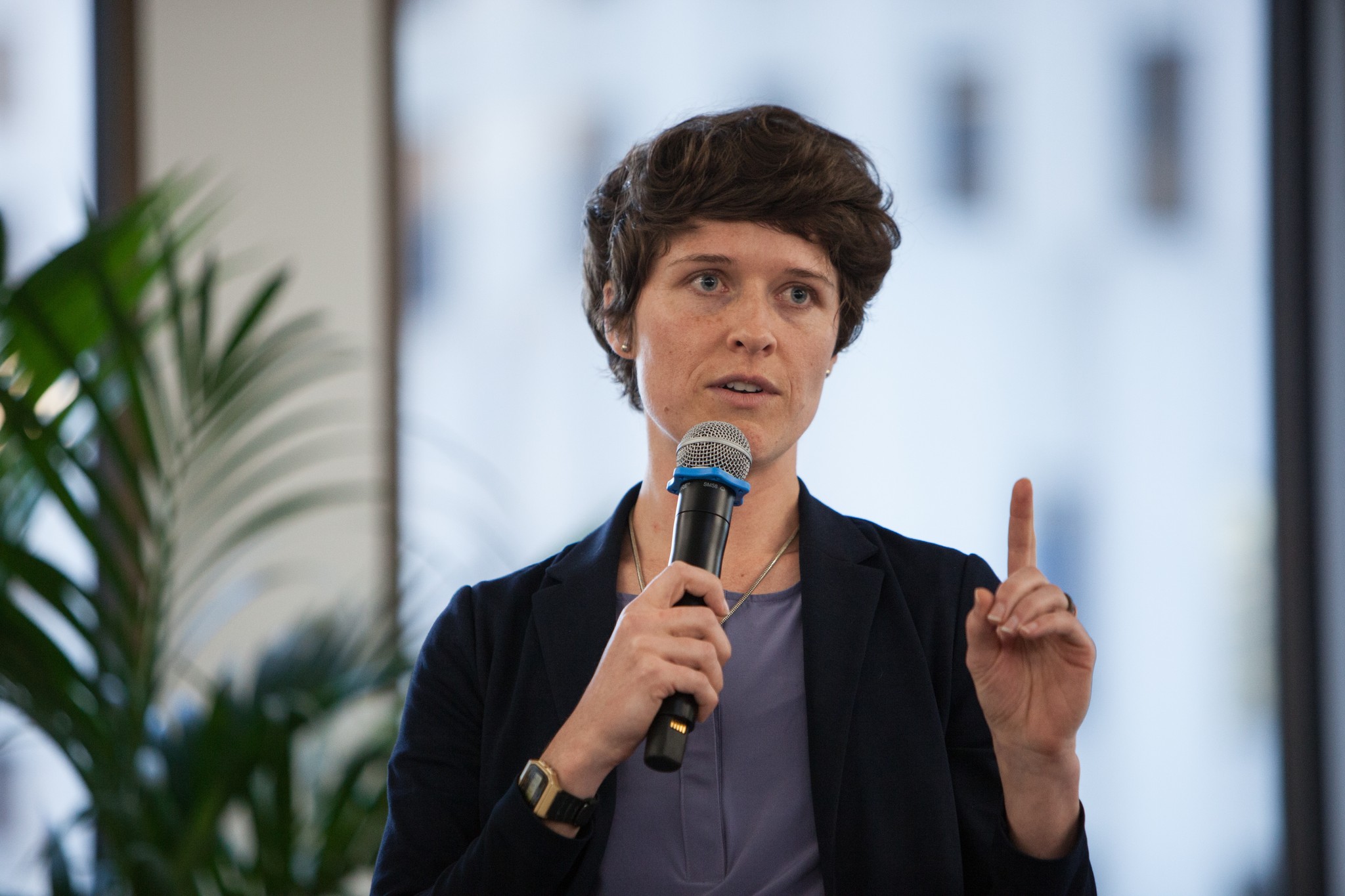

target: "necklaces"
[628,502,800,639]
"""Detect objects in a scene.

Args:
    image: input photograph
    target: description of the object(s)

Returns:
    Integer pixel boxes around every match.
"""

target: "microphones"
[642,420,754,772]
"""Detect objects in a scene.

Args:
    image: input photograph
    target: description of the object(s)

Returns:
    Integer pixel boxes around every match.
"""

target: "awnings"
[1064,592,1077,616]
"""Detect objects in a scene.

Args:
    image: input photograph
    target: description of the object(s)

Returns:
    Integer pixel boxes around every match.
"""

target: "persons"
[376,101,1097,895]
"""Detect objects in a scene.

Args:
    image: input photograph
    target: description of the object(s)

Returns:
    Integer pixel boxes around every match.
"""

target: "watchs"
[520,756,598,830]
